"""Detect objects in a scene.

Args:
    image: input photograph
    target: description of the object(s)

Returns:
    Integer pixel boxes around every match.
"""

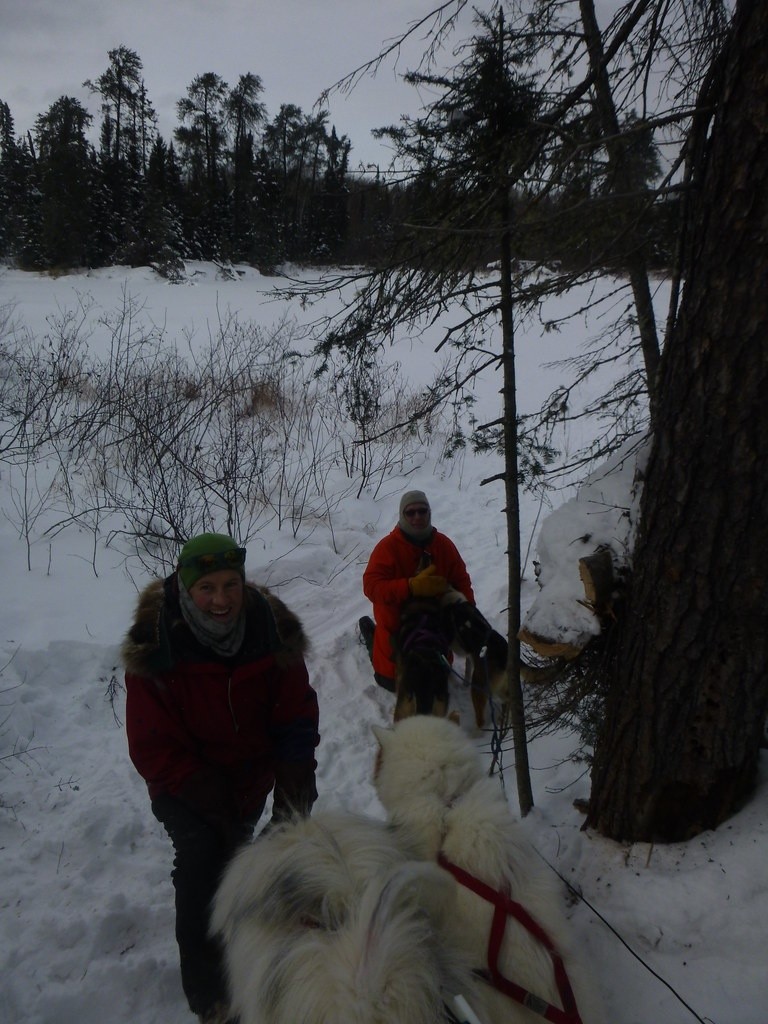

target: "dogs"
[206,708,609,1024]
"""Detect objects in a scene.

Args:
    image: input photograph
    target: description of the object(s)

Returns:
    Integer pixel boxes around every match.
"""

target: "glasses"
[404,508,428,517]
[179,548,246,574]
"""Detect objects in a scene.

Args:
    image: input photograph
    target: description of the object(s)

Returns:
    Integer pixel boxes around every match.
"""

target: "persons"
[122,533,320,1024]
[363,491,476,680]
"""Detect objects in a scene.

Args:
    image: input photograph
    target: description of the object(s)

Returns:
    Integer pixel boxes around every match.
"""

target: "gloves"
[408,565,449,598]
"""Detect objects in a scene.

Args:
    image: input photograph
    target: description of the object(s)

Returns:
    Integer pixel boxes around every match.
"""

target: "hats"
[399,490,432,539]
[178,533,246,591]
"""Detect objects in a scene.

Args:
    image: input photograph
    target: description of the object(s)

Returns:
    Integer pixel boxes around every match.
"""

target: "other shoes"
[193,996,239,1024]
